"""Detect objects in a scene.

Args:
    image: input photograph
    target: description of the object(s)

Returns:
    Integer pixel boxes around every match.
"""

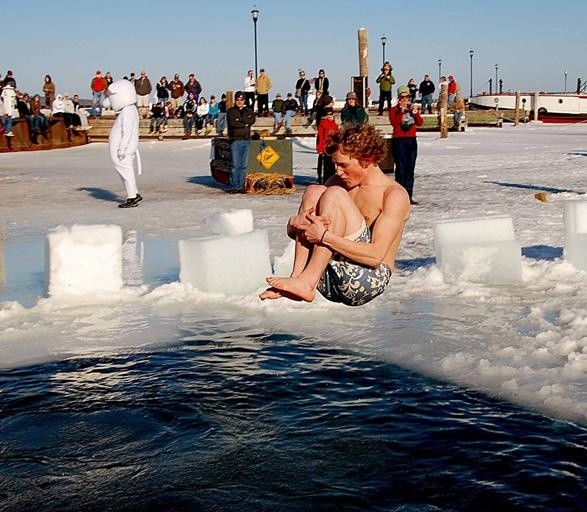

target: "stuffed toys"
[103,79,143,208]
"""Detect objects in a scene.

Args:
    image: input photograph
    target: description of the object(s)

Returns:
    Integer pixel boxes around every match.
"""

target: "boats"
[502,109,531,121]
[415,113,468,131]
[467,110,504,127]
[467,92,587,124]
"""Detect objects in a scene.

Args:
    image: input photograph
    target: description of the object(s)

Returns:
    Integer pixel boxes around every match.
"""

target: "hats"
[397,85,410,96]
[235,91,244,98]
[346,92,357,100]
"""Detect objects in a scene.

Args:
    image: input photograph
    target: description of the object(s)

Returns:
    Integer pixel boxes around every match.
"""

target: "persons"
[316,96,339,185]
[303,69,334,129]
[448,96,463,131]
[386,86,423,206]
[340,92,369,126]
[376,63,395,116]
[91,70,202,119]
[407,75,456,114]
[296,71,310,117]
[259,121,412,306]
[244,69,272,117]
[0,71,94,136]
[227,91,255,194]
[149,93,226,135]
[272,93,298,133]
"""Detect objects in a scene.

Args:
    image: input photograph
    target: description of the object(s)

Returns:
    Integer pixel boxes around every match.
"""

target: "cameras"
[407,102,412,106]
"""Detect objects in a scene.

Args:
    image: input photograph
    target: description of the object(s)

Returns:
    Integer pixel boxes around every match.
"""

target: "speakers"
[351,77,367,107]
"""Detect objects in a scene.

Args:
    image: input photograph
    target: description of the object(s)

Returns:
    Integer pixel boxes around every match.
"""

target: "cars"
[333,99,347,112]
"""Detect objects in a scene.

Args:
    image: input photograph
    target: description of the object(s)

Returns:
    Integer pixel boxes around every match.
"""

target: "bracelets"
[321,230,328,243]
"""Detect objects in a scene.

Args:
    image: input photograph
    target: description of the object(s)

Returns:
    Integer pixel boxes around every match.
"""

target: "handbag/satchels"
[295,89,302,97]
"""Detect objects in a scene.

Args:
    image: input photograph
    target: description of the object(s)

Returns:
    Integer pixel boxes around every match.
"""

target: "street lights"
[467,49,475,97]
[249,4,260,114]
[380,33,386,66]
[437,58,443,82]
[563,70,568,93]
[493,63,499,94]
